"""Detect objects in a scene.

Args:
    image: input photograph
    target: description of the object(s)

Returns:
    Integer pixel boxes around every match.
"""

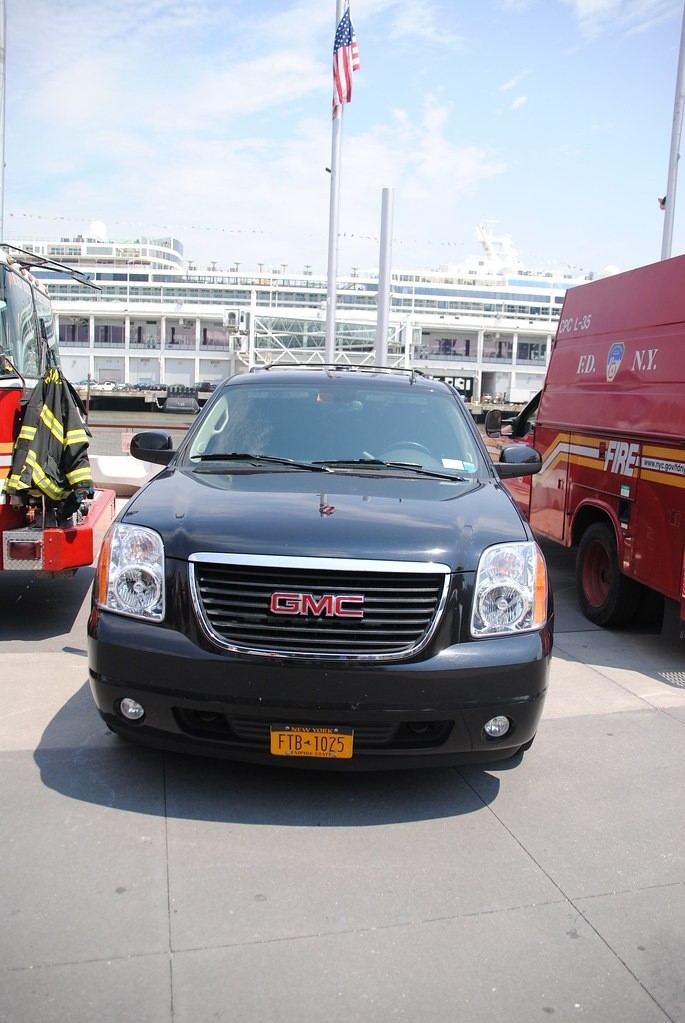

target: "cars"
[67,379,217,393]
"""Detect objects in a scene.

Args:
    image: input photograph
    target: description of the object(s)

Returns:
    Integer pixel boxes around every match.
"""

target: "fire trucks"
[0,242,117,576]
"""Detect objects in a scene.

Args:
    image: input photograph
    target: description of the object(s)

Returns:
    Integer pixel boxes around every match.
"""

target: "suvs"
[84,361,554,769]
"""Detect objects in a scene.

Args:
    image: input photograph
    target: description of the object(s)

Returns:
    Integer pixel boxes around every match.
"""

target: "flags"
[331,5,362,118]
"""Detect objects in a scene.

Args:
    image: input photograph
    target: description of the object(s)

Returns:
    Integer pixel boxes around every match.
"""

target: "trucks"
[480,254,685,632]
